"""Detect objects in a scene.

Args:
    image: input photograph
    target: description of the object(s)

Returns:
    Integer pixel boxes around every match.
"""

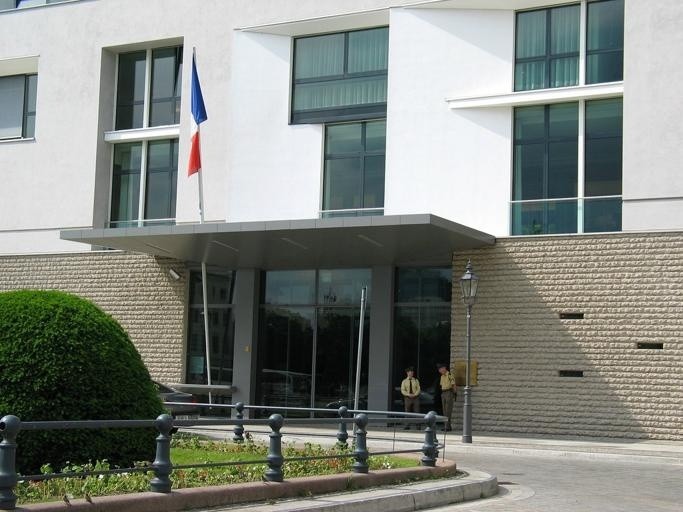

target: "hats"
[436,363,448,369]
[404,366,416,371]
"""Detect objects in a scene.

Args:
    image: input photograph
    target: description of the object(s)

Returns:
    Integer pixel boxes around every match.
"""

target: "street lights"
[458,257,476,444]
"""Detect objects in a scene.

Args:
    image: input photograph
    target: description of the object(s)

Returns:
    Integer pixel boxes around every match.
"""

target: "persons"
[435,361,455,430]
[400,365,421,430]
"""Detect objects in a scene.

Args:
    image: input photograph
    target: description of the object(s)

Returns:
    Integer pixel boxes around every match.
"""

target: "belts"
[442,389,451,392]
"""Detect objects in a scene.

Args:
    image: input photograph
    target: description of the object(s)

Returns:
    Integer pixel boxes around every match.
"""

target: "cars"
[150,378,200,435]
[324,385,434,418]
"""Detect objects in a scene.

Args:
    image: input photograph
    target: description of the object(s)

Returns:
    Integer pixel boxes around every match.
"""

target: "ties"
[410,379,411,393]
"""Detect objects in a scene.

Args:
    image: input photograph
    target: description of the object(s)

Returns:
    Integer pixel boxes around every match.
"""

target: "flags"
[186,54,207,176]
